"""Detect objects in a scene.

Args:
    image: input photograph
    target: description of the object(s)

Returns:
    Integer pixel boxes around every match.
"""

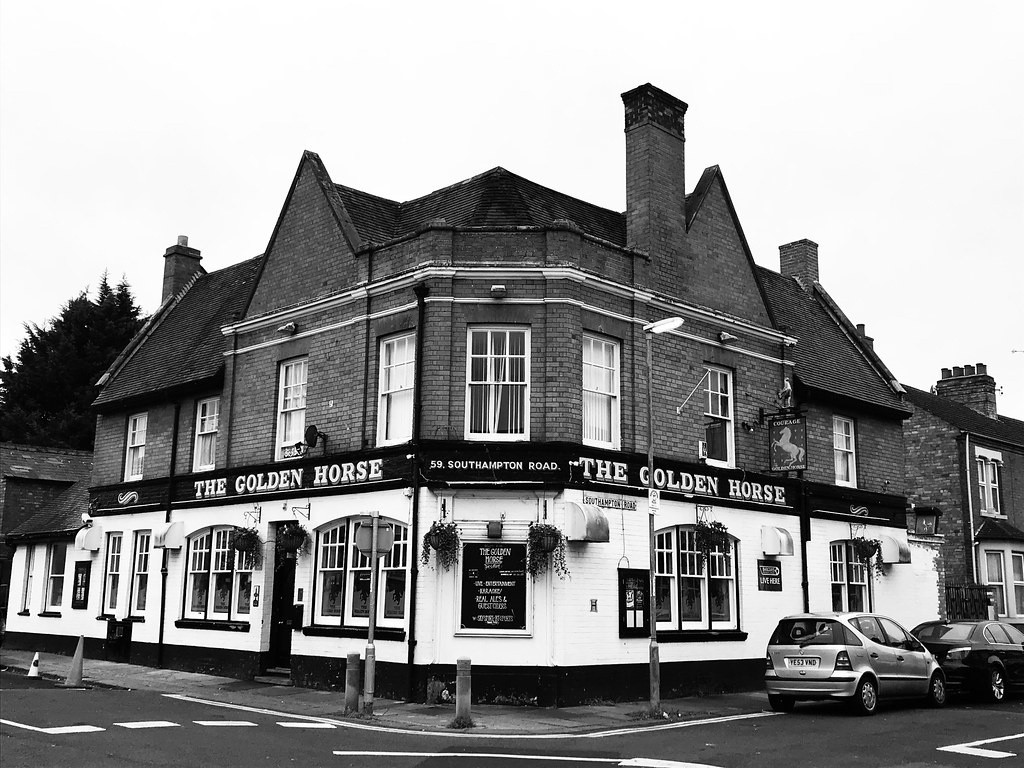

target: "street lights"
[642,316,685,713]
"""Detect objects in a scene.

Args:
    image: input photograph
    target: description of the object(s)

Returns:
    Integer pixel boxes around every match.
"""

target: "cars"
[903,618,1024,705]
[762,611,947,717]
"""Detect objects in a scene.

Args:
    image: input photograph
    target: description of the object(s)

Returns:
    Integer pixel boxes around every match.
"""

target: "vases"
[285,551,295,559]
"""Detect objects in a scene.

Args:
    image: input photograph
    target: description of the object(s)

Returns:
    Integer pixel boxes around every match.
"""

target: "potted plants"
[420,520,462,571]
[695,521,732,570]
[525,522,573,582]
[853,536,887,581]
[228,525,264,568]
[486,514,507,538]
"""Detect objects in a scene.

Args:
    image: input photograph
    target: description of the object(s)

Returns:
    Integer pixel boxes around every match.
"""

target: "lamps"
[277,321,297,334]
[491,284,507,300]
[719,331,740,344]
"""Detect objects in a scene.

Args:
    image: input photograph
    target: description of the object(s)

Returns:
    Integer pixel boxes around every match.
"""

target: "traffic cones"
[54,635,93,690]
[22,649,43,681]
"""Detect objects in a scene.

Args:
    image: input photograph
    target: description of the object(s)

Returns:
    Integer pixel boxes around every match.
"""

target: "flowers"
[275,524,311,560]
